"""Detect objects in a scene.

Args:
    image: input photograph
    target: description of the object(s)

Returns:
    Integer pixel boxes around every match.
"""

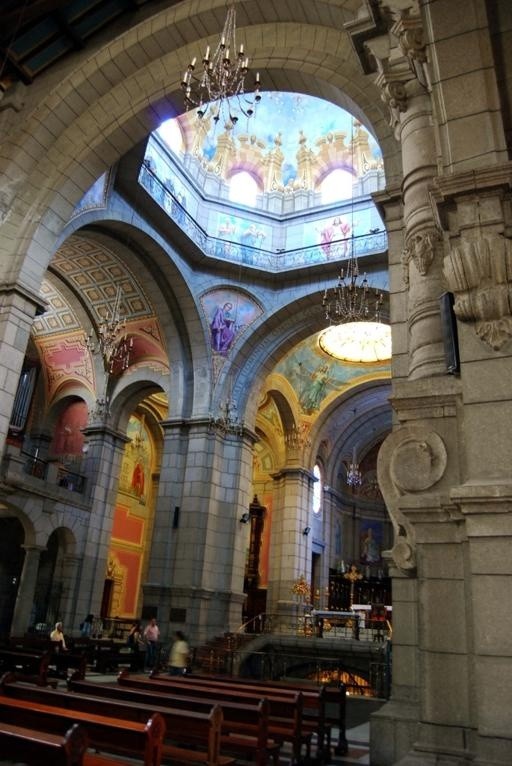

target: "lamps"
[179,4,263,133]
[83,266,138,375]
[130,414,149,458]
[320,105,384,329]
[346,443,363,486]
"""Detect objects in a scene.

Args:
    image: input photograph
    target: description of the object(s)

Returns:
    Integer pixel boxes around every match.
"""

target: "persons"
[126,623,146,673]
[140,150,158,191]
[78,614,94,638]
[208,301,239,354]
[156,171,177,207]
[313,215,361,262]
[48,621,71,654]
[360,528,382,565]
[334,519,343,555]
[166,629,193,677]
[240,224,266,264]
[172,183,188,227]
[92,615,104,635]
[214,217,239,264]
[143,618,161,674]
[131,454,146,499]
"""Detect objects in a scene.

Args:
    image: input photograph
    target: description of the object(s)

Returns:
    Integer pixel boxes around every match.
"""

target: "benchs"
[0,671,348,764]
[2,628,148,676]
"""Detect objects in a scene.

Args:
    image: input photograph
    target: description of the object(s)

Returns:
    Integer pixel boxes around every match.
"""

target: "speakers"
[439,291,460,375]
